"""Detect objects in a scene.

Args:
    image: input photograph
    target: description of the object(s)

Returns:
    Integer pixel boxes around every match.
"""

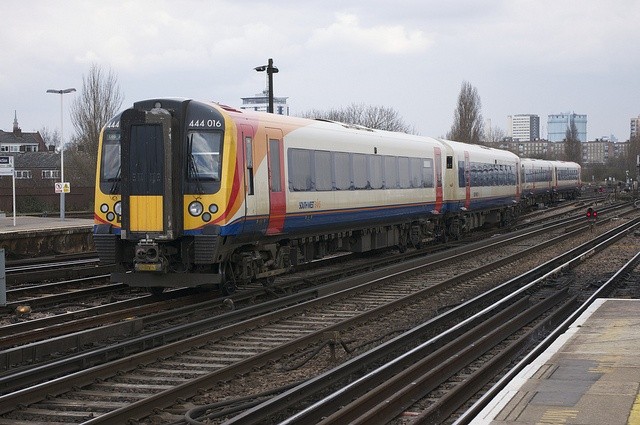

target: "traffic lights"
[587,208,598,227]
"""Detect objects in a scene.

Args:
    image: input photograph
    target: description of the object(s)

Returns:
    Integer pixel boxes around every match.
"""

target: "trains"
[520,157,583,210]
[93,99,520,296]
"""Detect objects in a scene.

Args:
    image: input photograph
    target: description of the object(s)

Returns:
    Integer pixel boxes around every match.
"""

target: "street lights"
[255,58,279,112]
[45,87,75,218]
